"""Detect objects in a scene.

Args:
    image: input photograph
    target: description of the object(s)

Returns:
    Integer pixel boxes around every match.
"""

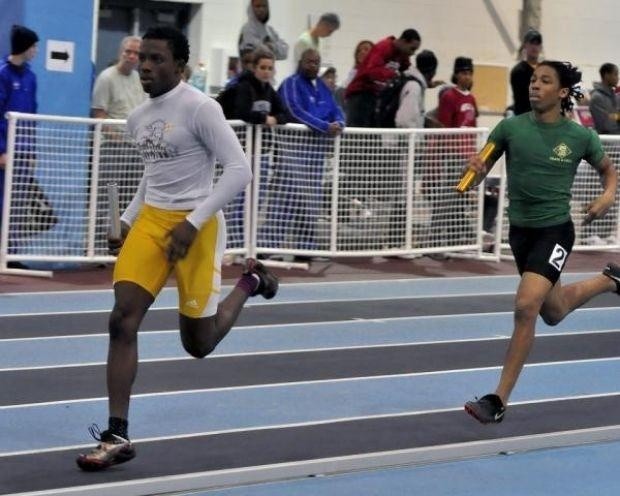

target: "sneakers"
[77,423,135,470]
[465,393,507,425]
[242,258,277,300]
[603,263,620,295]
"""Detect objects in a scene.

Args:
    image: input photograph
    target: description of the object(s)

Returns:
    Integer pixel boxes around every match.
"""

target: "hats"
[524,31,541,43]
[11,24,38,55]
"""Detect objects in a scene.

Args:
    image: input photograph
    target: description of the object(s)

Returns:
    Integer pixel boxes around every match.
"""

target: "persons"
[0,23,58,271]
[511,30,543,116]
[590,64,619,135]
[90,35,147,255]
[214,0,345,271]
[75,28,277,467]
[336,28,478,263]
[458,62,620,425]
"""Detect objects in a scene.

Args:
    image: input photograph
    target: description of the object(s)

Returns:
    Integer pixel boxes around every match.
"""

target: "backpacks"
[377,76,422,127]
